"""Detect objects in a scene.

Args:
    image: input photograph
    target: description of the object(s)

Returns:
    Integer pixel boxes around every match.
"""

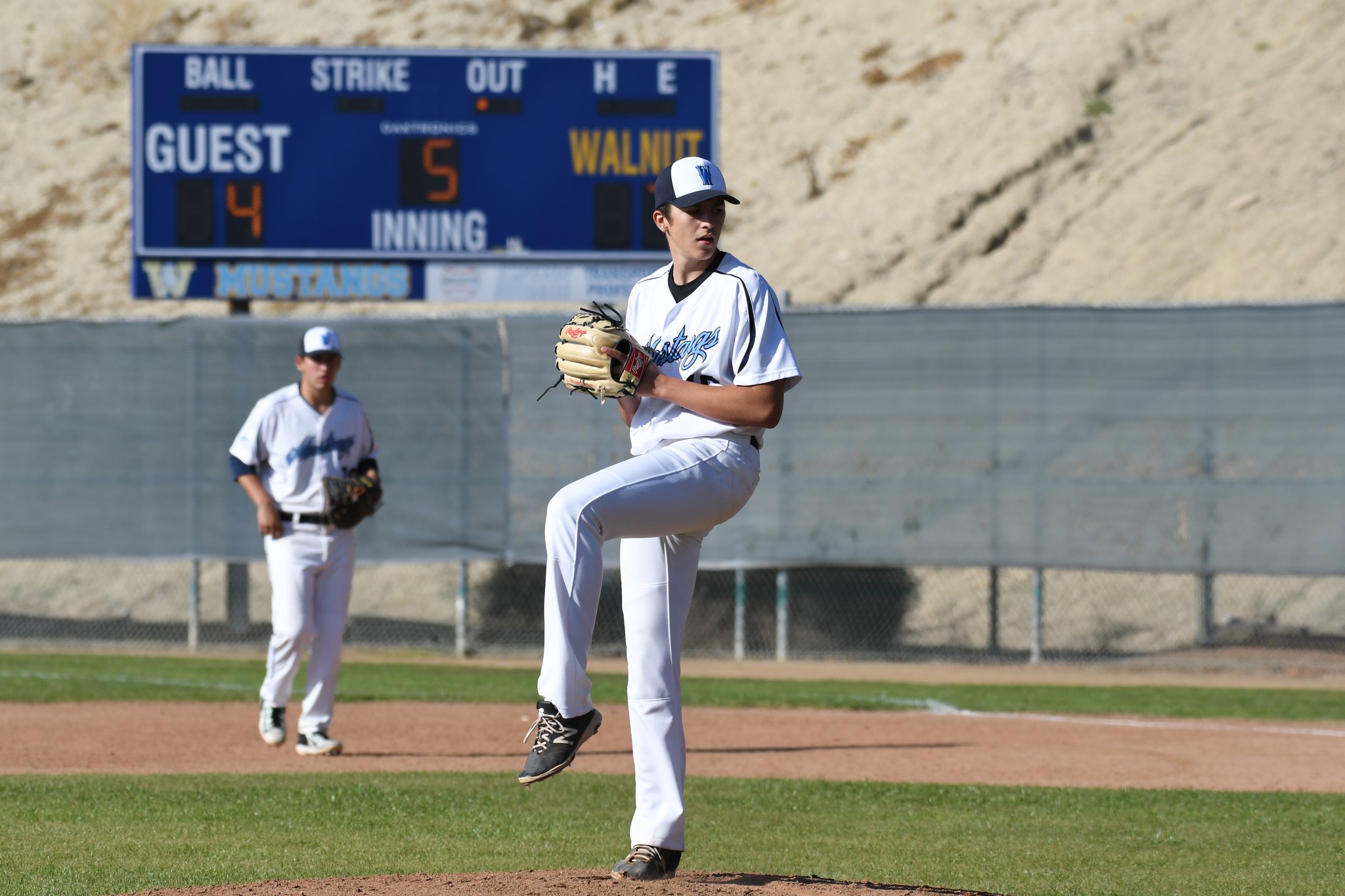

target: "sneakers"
[518,700,602,788]
[295,732,343,756]
[258,701,286,747]
[610,845,682,882]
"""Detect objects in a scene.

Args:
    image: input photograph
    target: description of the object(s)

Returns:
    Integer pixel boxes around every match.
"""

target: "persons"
[517,155,804,881]
[227,326,384,756]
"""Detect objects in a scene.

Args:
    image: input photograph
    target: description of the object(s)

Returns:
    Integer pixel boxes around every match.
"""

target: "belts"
[750,434,759,451]
[279,510,330,526]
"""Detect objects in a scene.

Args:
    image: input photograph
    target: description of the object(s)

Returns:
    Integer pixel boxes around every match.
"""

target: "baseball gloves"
[323,476,382,529]
[554,312,654,399]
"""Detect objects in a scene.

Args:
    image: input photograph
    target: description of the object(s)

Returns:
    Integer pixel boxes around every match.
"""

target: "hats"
[299,325,343,361]
[654,156,740,211]
